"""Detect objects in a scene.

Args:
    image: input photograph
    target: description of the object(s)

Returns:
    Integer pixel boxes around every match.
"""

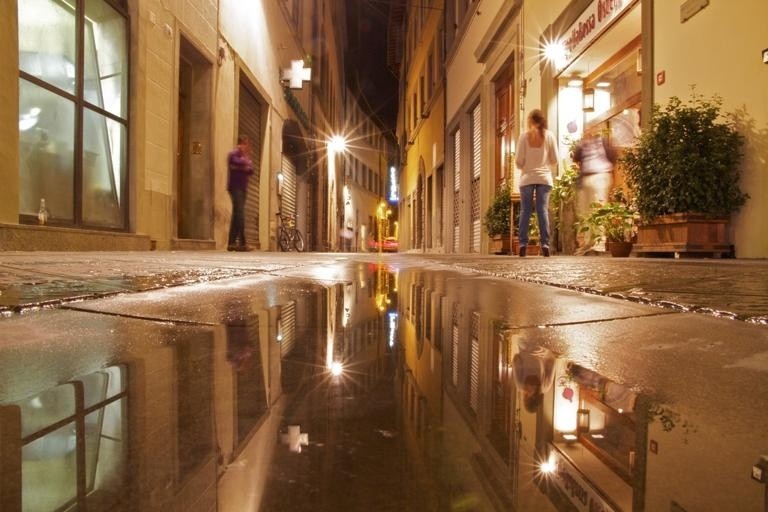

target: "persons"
[225,135,256,252]
[225,318,252,375]
[515,108,559,258]
[572,122,617,255]
[344,227,354,252]
[512,340,555,413]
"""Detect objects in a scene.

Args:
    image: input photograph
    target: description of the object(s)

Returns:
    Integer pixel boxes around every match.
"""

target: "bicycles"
[276,212,304,252]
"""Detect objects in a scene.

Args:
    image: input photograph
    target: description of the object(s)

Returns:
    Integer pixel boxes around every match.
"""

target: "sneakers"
[227,244,255,251]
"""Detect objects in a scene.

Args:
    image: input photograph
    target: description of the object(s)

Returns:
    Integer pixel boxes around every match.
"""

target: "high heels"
[542,247,549,257]
[520,246,526,256]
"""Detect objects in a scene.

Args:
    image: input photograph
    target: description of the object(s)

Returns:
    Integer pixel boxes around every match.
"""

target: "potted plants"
[480,175,542,257]
[550,82,750,254]
